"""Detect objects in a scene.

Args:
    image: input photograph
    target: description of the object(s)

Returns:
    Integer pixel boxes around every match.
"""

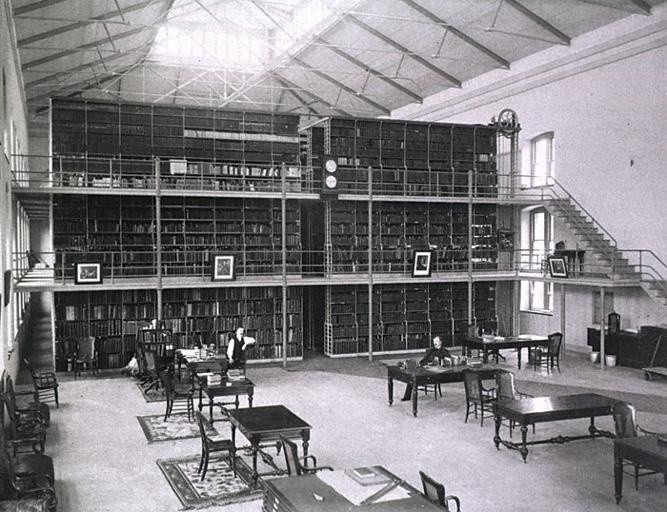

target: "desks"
[195,373,254,427]
[614,435,667,505]
[387,364,501,418]
[554,249,584,276]
[493,392,621,463]
[263,466,448,512]
[228,405,312,490]
[466,335,554,369]
[177,348,226,387]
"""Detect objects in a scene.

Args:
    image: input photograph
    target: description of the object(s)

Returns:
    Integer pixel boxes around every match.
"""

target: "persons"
[402,336,449,401]
[217,259,231,274]
[227,327,255,368]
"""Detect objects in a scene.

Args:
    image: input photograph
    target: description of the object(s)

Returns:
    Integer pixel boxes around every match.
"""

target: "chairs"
[74,336,97,379]
[133,330,195,421]
[0,357,59,512]
[496,373,536,434]
[463,369,498,428]
[612,401,667,490]
[197,413,236,480]
[281,434,334,476]
[535,332,563,373]
[420,470,460,512]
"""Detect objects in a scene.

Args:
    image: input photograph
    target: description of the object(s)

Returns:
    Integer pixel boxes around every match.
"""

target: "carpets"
[156,455,270,509]
[134,412,221,445]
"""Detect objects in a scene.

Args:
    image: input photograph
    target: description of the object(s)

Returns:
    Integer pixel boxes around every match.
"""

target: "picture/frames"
[548,259,568,277]
